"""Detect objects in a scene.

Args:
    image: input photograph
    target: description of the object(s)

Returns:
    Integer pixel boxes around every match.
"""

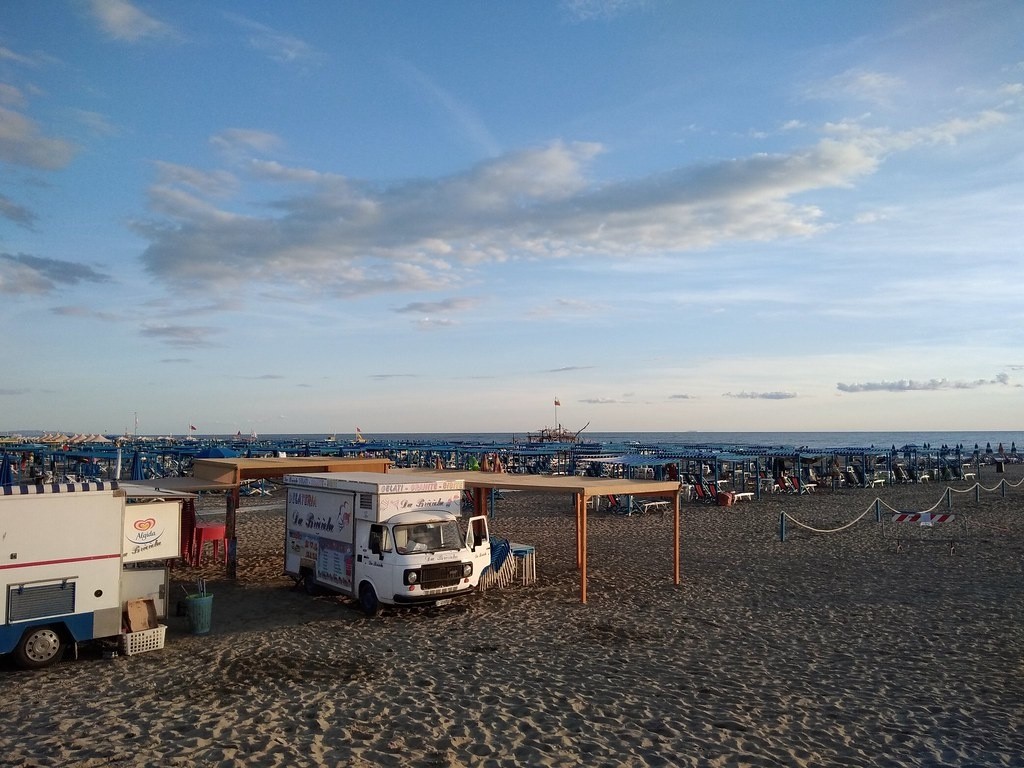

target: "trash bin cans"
[184,591,215,635]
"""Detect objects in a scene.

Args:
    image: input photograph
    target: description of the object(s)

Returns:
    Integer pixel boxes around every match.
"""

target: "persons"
[534,455,841,482]
[0,451,35,475]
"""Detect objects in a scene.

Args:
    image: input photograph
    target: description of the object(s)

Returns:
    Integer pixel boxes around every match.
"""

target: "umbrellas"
[195,447,242,458]
[130,448,148,503]
[868,442,1018,464]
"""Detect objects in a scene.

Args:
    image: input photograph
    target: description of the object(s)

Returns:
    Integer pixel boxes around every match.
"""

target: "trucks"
[0,479,199,670]
[282,470,491,618]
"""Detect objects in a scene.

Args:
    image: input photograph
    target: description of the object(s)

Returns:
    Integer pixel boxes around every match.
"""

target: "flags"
[357,427,360,432]
[191,425,196,430]
[555,400,560,406]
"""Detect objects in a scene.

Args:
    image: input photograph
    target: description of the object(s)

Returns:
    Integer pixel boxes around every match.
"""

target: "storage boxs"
[118,623,168,656]
[126,599,159,633]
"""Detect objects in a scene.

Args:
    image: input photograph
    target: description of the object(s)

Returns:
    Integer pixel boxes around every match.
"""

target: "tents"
[1,432,114,444]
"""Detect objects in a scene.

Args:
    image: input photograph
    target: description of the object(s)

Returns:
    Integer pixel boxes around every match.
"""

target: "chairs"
[10,454,1019,593]
